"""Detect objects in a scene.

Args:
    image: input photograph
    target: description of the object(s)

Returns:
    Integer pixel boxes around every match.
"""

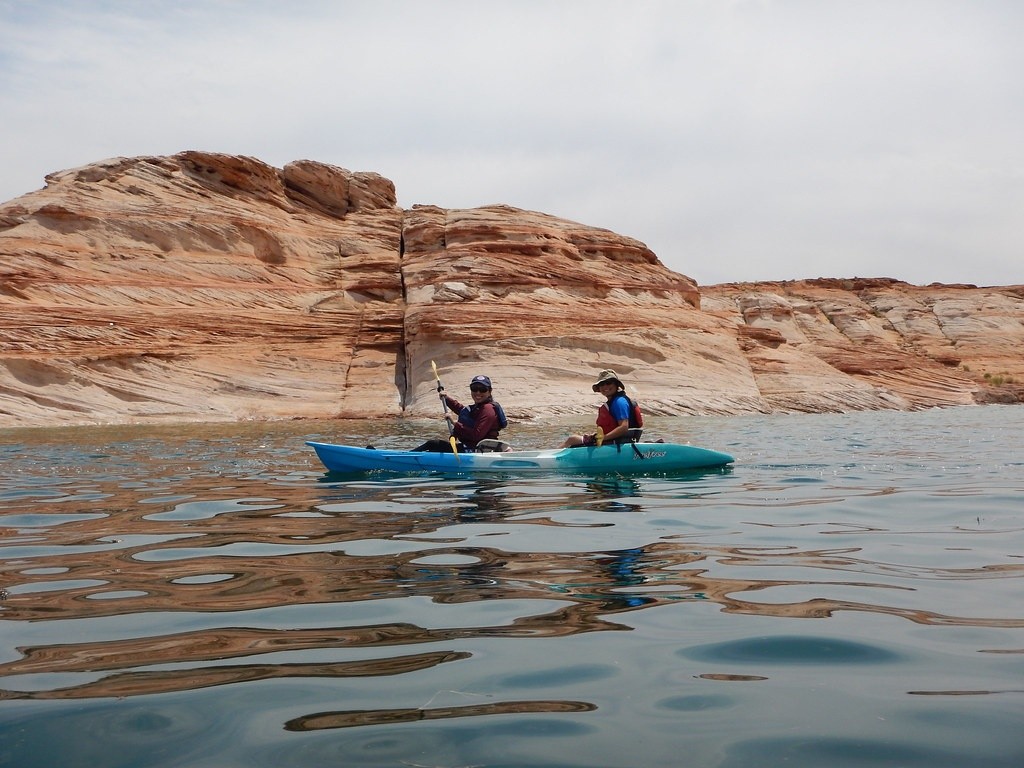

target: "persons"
[365,375,508,451]
[559,369,643,447]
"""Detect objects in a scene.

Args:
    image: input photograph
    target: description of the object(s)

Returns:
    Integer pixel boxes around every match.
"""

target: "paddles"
[593,426,605,448]
[429,357,461,456]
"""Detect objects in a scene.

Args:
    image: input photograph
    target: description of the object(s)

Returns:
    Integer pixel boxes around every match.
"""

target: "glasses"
[470,386,490,393]
[599,381,616,386]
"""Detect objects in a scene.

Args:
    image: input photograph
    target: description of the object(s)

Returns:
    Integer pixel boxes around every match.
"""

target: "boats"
[304,441,737,477]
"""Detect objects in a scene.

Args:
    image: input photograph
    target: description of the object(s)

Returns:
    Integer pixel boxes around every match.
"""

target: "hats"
[592,369,625,392]
[470,375,491,388]
